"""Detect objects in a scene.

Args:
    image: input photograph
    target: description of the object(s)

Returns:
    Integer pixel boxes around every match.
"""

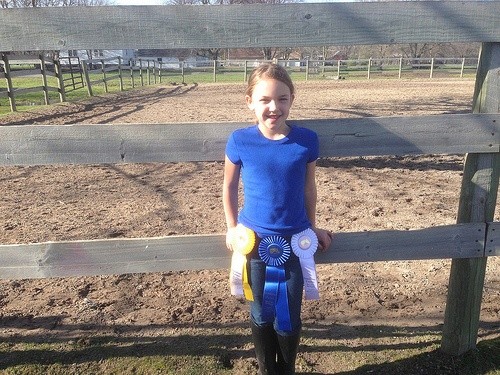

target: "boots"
[249,321,303,375]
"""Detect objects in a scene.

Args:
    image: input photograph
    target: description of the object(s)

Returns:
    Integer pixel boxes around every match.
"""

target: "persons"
[222,64,334,375]
